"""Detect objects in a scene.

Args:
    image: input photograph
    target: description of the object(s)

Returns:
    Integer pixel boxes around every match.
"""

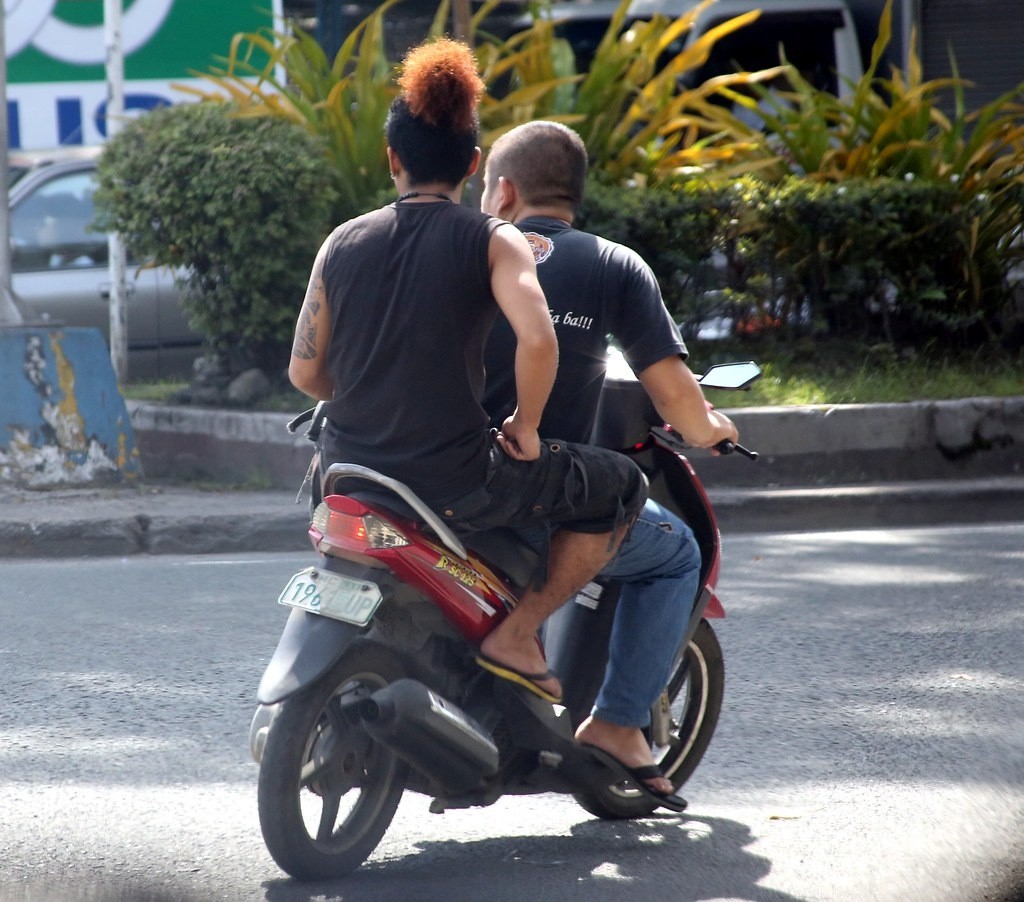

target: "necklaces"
[397,192,450,202]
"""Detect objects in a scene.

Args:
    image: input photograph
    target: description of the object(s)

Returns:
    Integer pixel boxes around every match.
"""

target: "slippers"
[475,652,566,705]
[590,745,693,809]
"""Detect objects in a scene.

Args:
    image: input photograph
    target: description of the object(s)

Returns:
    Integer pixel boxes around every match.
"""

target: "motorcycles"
[245,357,766,884]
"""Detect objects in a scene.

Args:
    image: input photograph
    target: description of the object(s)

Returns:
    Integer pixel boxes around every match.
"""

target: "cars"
[5,152,210,385]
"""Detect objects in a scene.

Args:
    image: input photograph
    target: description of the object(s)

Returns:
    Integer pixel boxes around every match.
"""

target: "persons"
[480,117,738,814]
[287,37,650,704]
